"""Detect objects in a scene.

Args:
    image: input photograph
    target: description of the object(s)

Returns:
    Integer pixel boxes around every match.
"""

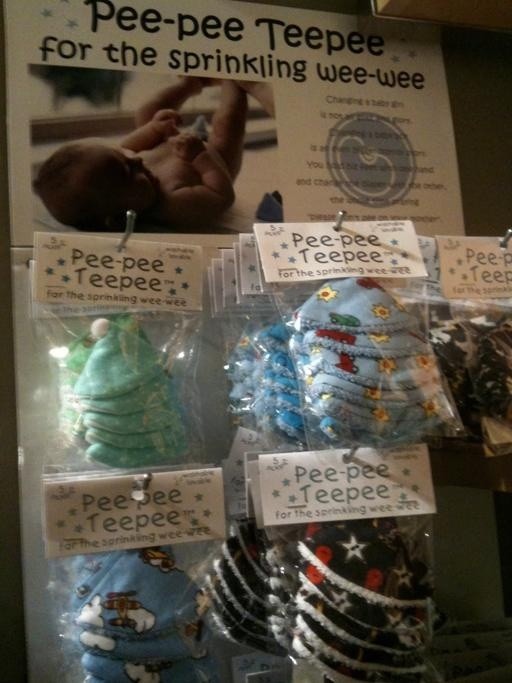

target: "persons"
[34,73,247,234]
